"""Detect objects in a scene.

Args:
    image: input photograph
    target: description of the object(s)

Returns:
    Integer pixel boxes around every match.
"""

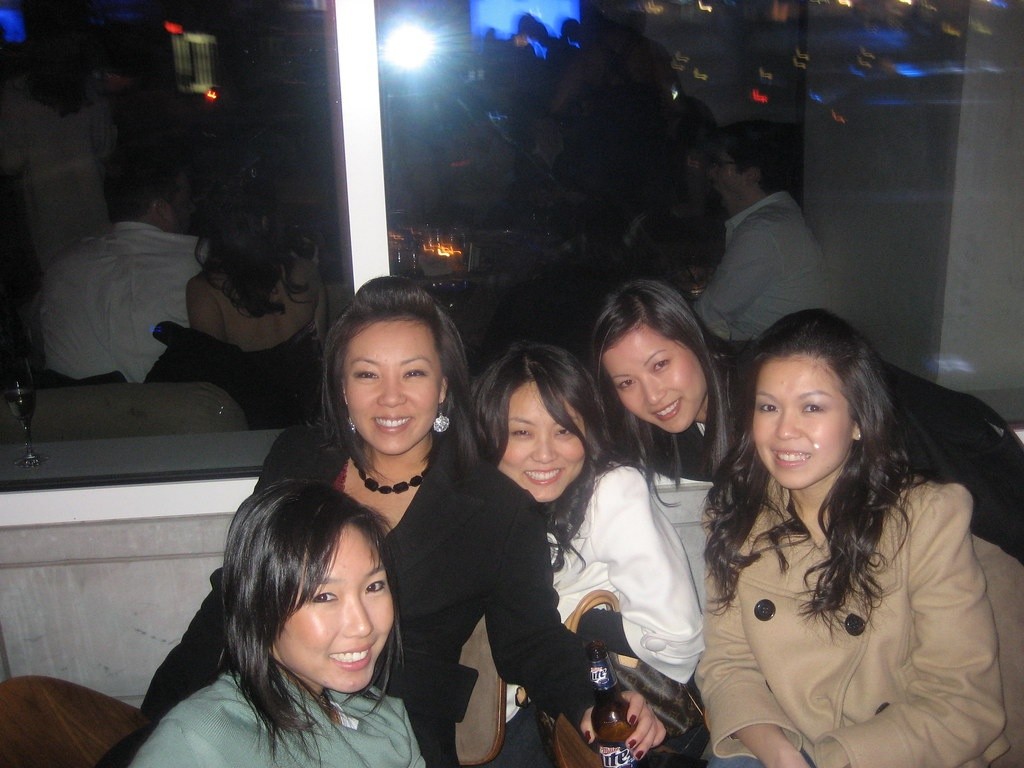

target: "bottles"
[586,640,635,768]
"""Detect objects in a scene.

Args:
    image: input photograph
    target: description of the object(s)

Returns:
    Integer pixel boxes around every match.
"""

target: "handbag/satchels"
[539,589,706,768]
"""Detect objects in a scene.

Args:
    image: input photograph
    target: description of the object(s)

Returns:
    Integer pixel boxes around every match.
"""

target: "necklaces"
[347,449,431,495]
[319,691,331,718]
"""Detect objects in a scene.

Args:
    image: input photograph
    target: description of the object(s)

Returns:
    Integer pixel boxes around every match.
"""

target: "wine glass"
[0,356,51,468]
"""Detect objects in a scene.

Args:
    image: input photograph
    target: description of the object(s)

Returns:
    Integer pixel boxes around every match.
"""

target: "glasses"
[712,155,758,171]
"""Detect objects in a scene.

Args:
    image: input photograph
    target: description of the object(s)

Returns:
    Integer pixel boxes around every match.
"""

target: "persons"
[587,275,1024,568]
[126,475,427,768]
[90,274,670,768]
[471,337,707,768]
[1,18,823,437]
[694,306,1010,768]
